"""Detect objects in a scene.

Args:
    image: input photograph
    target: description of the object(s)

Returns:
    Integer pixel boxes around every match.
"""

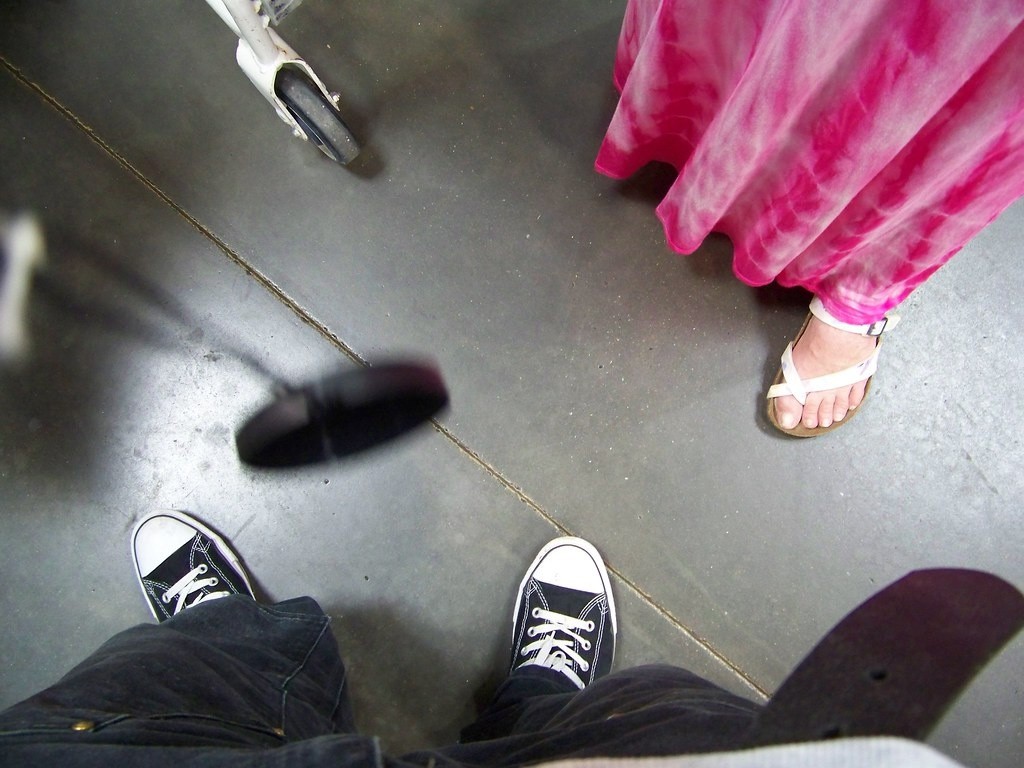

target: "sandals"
[763,298,902,437]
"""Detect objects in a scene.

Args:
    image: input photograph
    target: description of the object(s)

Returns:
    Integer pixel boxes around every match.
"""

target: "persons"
[0,508,1023,768]
[596,0,1024,438]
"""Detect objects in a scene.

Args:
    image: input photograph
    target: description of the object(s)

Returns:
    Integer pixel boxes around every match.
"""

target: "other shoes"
[131,509,256,624]
[508,536,617,693]
